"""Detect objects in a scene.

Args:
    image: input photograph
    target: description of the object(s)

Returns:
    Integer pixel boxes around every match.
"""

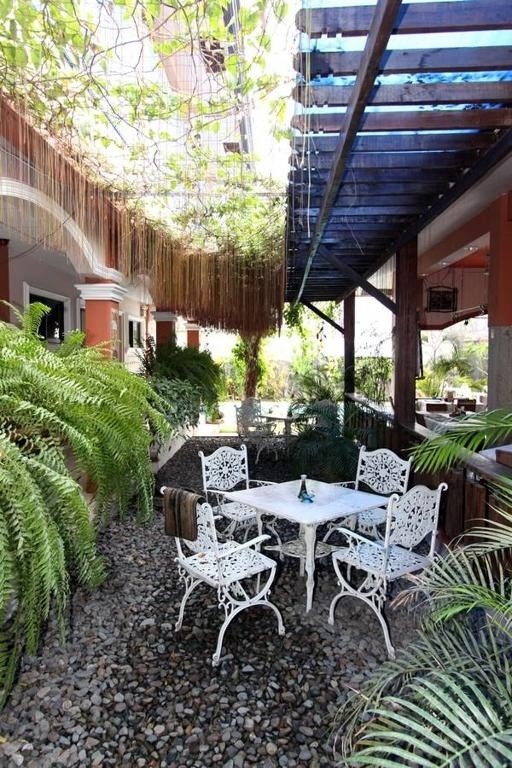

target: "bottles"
[297,475,309,499]
